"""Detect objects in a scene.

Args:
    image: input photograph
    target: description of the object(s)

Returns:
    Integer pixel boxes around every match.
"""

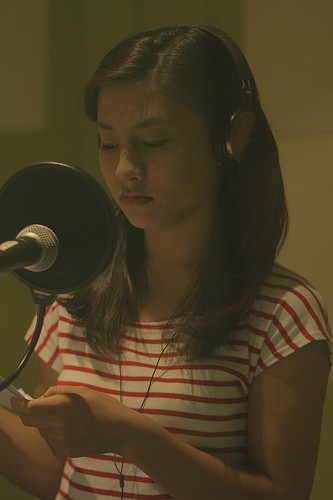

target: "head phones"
[196,24,262,167]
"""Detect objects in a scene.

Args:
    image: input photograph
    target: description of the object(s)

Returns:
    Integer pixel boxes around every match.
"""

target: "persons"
[0,26,333,500]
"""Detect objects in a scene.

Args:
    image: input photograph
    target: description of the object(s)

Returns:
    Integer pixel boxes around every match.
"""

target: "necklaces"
[113,294,198,500]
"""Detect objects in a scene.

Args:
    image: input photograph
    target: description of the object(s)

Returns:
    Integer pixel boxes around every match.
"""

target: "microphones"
[0,224,60,274]
